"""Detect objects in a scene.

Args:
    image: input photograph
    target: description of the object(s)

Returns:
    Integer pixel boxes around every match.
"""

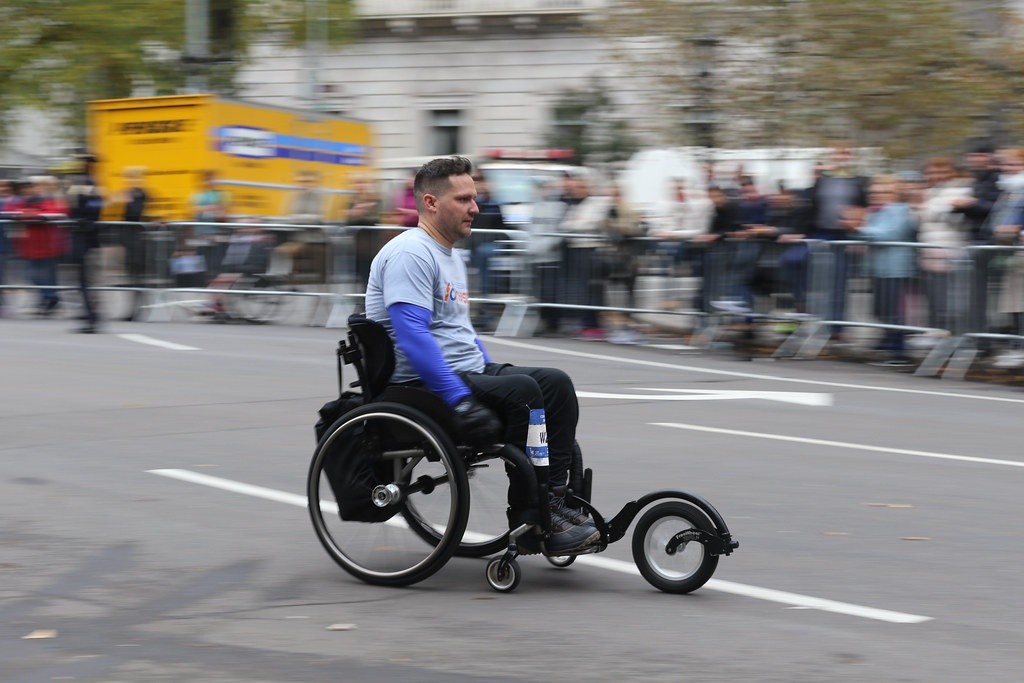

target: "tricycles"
[309,312,740,594]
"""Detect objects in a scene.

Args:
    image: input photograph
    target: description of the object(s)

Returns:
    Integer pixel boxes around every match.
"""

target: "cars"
[480,162,605,249]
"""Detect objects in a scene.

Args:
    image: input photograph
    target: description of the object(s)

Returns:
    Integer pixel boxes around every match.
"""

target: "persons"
[697,147,1024,370]
[365,155,609,553]
[469,176,503,325]
[344,173,419,282]
[529,171,645,341]
[191,170,232,273]
[0,151,144,332]
[292,171,332,290]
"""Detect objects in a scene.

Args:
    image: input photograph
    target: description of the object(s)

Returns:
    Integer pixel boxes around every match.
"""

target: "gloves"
[445,389,501,446]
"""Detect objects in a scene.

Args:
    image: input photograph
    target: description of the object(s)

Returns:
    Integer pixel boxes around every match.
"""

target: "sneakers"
[505,493,602,553]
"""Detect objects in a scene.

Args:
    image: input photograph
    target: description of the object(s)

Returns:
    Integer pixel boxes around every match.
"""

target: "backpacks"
[315,392,398,522]
